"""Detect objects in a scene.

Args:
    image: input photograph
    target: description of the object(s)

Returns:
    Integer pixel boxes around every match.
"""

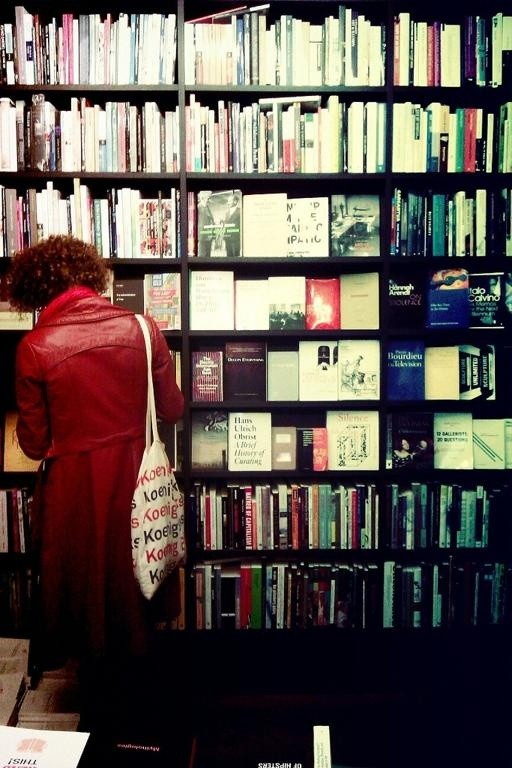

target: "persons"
[5,235,184,651]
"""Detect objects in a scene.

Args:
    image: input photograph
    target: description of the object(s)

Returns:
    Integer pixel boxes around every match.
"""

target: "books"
[153,349,182,470]
[183,3,382,471]
[387,11,512,468]
[1,487,44,629]
[0,5,179,332]
[0,723,332,768]
[189,480,512,629]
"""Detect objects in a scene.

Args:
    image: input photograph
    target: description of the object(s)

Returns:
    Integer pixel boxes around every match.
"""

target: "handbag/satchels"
[129,438,187,601]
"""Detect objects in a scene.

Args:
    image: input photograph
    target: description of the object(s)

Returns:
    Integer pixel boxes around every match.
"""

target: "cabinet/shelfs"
[1,0,512,655]
